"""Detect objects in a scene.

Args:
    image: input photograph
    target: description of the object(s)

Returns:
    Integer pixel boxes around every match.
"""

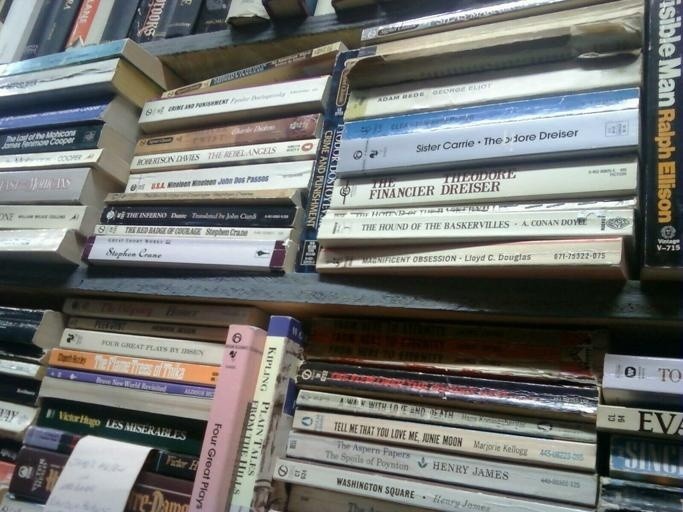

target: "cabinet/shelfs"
[1,0,683,512]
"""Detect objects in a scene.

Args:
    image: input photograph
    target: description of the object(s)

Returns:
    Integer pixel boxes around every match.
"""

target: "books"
[1,290,683,511]
[1,0,683,281]
